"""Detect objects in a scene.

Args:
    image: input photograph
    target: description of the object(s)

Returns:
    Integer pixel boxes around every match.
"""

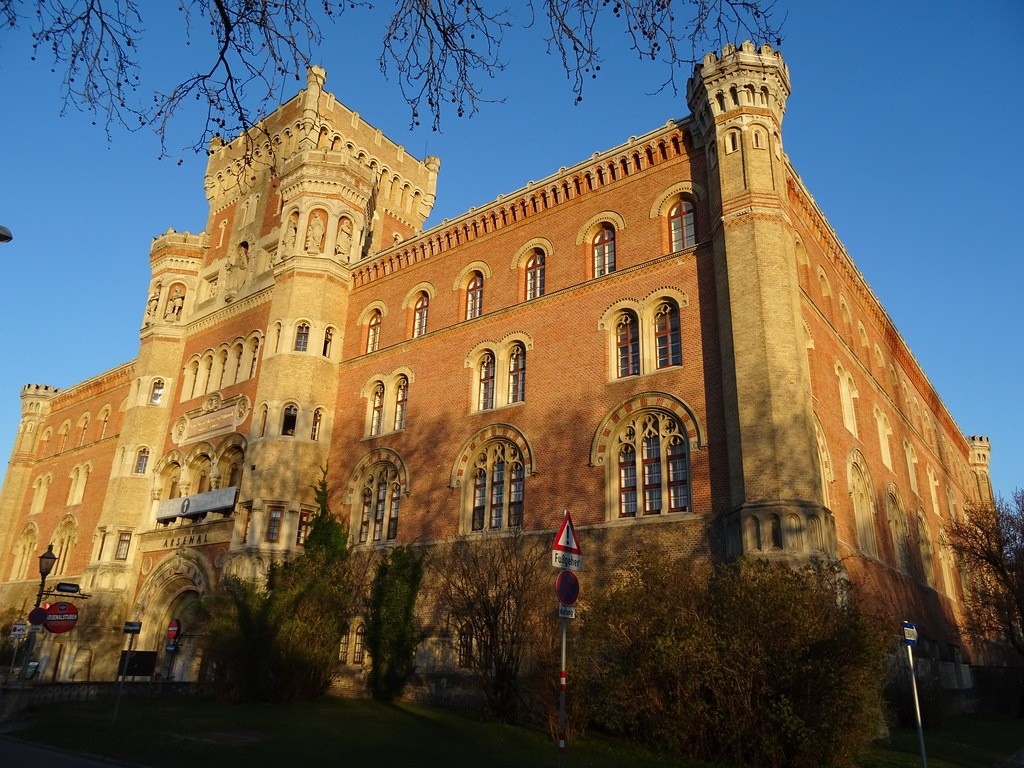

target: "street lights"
[14,541,60,680]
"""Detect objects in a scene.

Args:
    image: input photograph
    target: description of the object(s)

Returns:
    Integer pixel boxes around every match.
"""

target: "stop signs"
[43,601,78,635]
[167,620,181,638]
